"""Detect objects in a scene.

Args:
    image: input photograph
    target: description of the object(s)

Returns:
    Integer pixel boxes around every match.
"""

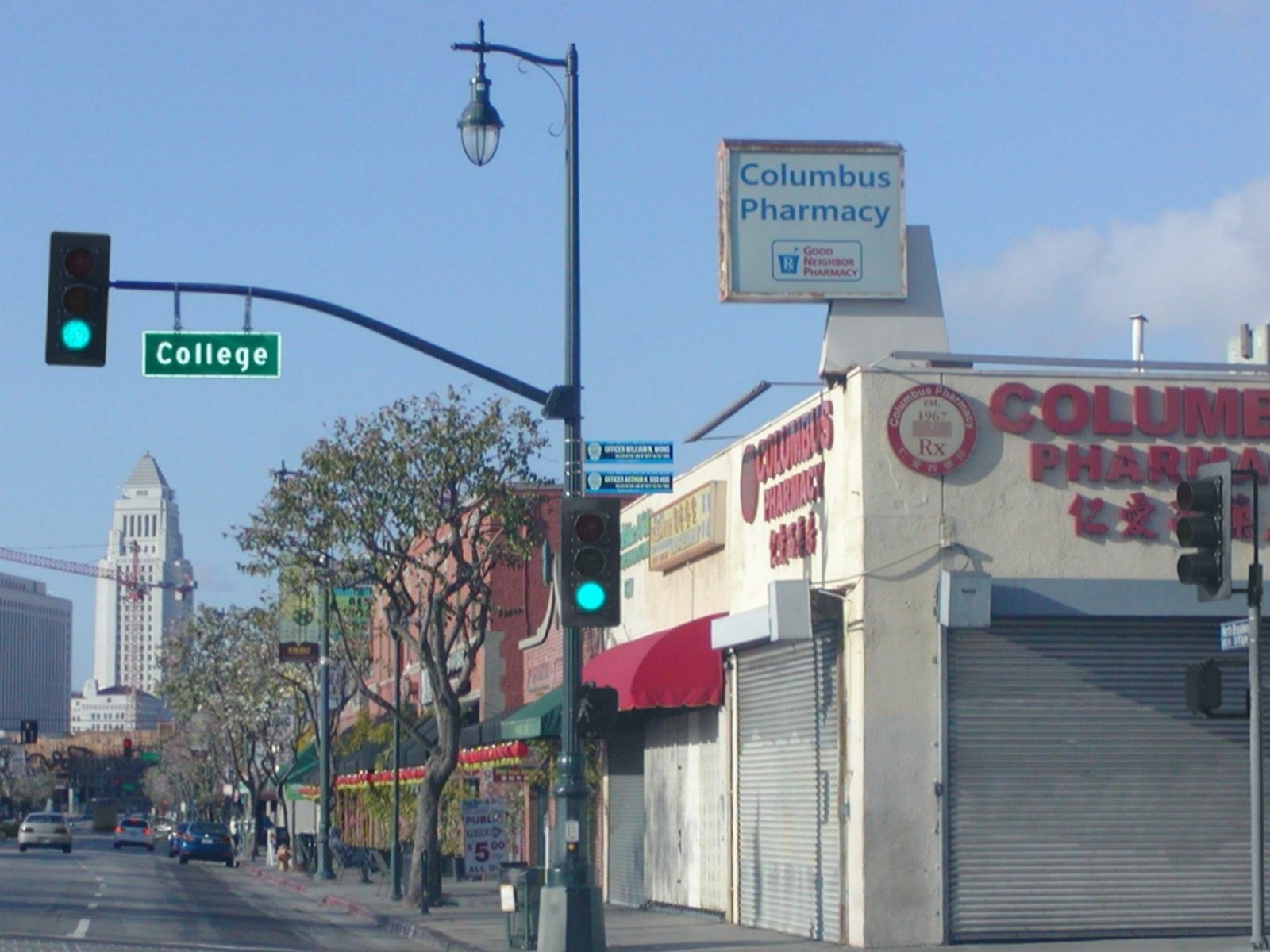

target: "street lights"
[443,15,613,952]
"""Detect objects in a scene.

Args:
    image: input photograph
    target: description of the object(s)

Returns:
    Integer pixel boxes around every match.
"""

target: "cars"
[179,819,236,869]
[166,824,189,858]
[151,815,177,840]
[15,810,75,854]
[112,818,155,851]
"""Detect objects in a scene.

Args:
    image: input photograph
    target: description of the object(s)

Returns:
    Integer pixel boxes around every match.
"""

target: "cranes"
[0,530,206,605]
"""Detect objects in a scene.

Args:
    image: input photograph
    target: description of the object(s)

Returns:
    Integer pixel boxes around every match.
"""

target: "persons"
[224,813,274,856]
[328,827,380,884]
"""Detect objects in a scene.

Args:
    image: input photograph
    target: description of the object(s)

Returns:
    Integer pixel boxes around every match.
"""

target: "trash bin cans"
[499,867,544,949]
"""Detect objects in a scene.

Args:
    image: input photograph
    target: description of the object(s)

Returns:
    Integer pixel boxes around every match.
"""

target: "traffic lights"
[45,230,120,368]
[560,488,620,631]
[1175,457,1241,601]
[120,739,134,760]
[1185,659,1222,711]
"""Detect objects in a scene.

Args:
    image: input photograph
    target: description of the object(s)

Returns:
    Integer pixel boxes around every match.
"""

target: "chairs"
[373,850,404,895]
[327,841,364,886]
[299,841,323,874]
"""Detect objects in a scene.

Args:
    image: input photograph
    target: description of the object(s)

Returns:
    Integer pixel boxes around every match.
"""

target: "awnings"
[580,612,730,711]
[272,700,478,780]
[461,683,592,747]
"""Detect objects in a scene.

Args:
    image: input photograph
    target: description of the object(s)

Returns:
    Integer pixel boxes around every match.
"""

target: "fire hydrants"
[275,843,292,871]
